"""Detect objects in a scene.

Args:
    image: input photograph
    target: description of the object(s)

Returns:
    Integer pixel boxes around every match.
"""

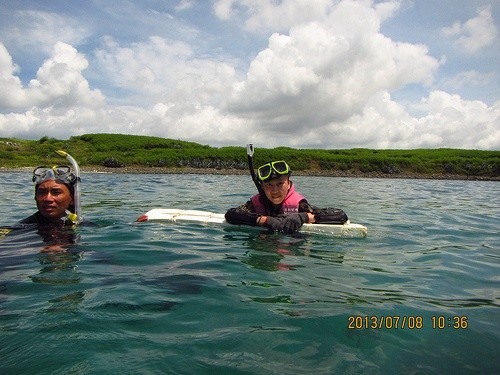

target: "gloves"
[279,212,309,234]
[265,214,290,231]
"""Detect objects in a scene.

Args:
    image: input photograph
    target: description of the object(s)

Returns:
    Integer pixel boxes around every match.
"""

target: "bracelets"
[258,215,263,225]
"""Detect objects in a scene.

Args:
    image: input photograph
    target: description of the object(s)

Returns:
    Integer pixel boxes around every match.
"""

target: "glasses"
[32,165,76,197]
[257,160,292,181]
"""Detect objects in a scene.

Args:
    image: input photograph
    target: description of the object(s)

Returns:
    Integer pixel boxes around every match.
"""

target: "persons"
[15,164,99,227]
[225,160,349,234]
